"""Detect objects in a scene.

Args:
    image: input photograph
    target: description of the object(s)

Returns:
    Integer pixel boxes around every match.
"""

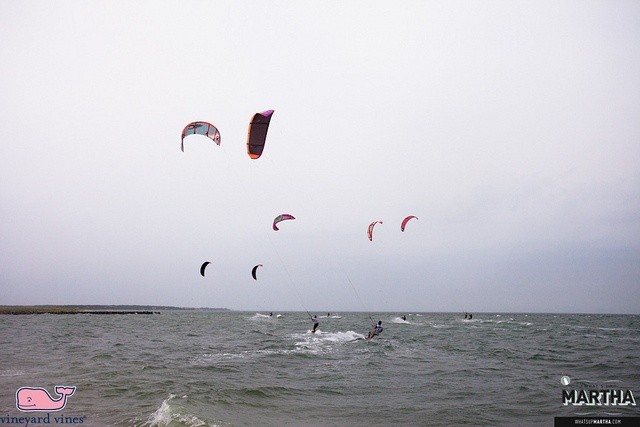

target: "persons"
[269,312,272,317]
[311,315,319,333]
[403,315,406,321]
[464,313,467,319]
[469,314,472,319]
[365,321,383,341]
